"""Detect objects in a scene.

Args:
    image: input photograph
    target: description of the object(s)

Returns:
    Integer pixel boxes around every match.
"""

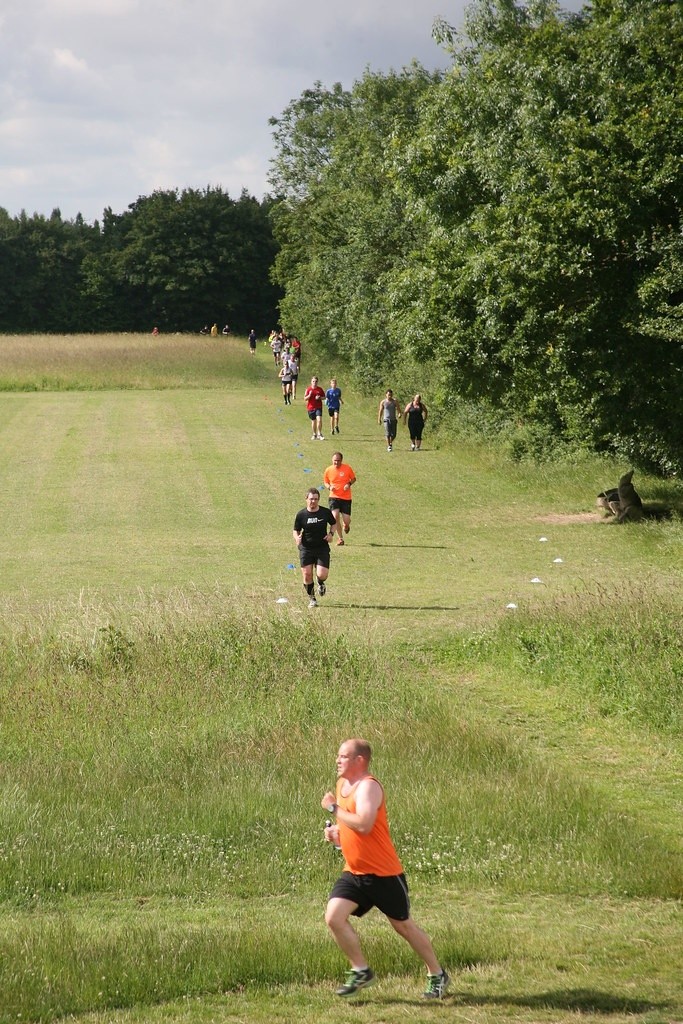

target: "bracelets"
[329,532,334,536]
[349,482,352,486]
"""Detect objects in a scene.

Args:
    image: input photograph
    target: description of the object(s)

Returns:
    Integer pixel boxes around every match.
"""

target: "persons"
[222,325,230,335]
[325,379,344,435]
[249,330,257,354]
[303,376,326,441]
[293,487,337,608]
[288,354,300,399]
[211,324,218,336]
[152,327,159,335]
[199,325,210,334]
[269,327,301,371]
[321,738,451,999]
[378,389,402,452]
[279,361,293,405]
[403,395,427,450]
[324,452,356,546]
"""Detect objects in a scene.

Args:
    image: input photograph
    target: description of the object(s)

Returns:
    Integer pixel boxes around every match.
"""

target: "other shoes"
[388,443,392,452]
[332,430,335,435]
[285,401,287,405]
[288,400,291,405]
[343,522,349,534]
[336,539,344,546]
[310,435,317,440]
[411,443,420,451]
[317,434,325,440]
[334,426,339,434]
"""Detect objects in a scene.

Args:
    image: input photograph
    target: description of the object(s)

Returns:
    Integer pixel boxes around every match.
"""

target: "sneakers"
[421,968,451,998]
[316,576,326,597]
[336,967,375,997]
[307,601,316,609]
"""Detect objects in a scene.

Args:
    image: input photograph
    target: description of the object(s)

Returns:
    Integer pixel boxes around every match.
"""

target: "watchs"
[328,803,338,813]
